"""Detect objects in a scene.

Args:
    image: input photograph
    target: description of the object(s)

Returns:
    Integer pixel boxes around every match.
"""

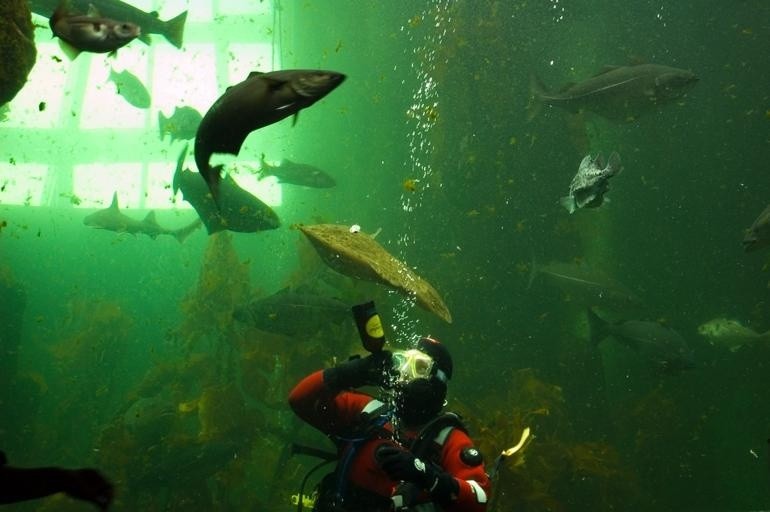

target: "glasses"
[395,349,436,381]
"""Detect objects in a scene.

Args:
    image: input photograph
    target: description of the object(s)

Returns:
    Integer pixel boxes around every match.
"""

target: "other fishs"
[158,65,455,339]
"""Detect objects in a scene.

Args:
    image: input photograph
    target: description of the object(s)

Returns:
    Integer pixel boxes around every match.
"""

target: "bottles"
[348,297,388,359]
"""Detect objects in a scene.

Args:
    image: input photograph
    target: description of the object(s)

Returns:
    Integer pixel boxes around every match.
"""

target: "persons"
[1,442,113,512]
[288,339,489,510]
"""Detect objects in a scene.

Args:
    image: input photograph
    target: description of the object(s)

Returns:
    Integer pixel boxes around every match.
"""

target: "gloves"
[376,446,451,498]
[323,351,394,393]
